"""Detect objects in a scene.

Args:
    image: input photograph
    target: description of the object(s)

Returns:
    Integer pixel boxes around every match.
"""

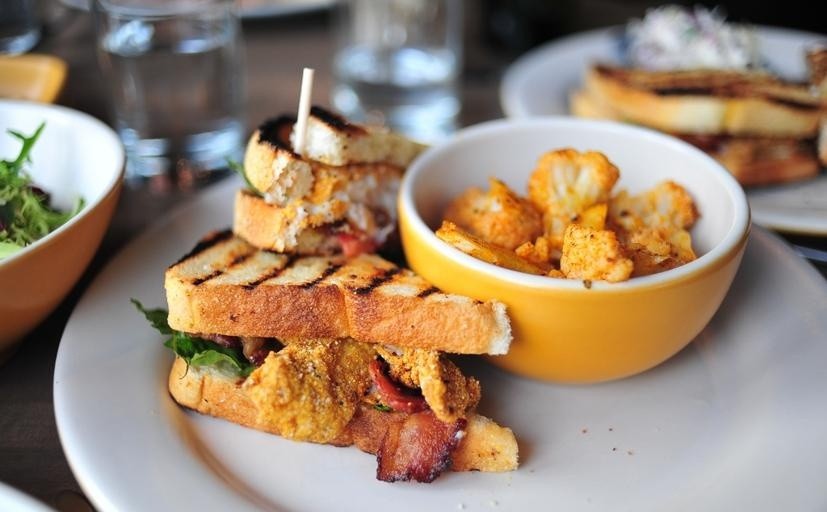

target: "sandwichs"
[163,226,520,485]
[233,105,431,258]
[568,63,827,186]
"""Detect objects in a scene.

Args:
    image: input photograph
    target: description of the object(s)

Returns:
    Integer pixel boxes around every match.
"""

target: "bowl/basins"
[0,97,126,352]
[395,115,752,386]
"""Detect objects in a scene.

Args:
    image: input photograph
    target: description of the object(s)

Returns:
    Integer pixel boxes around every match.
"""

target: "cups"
[88,0,249,183]
[323,0,468,149]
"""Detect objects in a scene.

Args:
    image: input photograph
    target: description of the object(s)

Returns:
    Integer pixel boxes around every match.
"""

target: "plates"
[50,169,827,511]
[498,20,827,236]
